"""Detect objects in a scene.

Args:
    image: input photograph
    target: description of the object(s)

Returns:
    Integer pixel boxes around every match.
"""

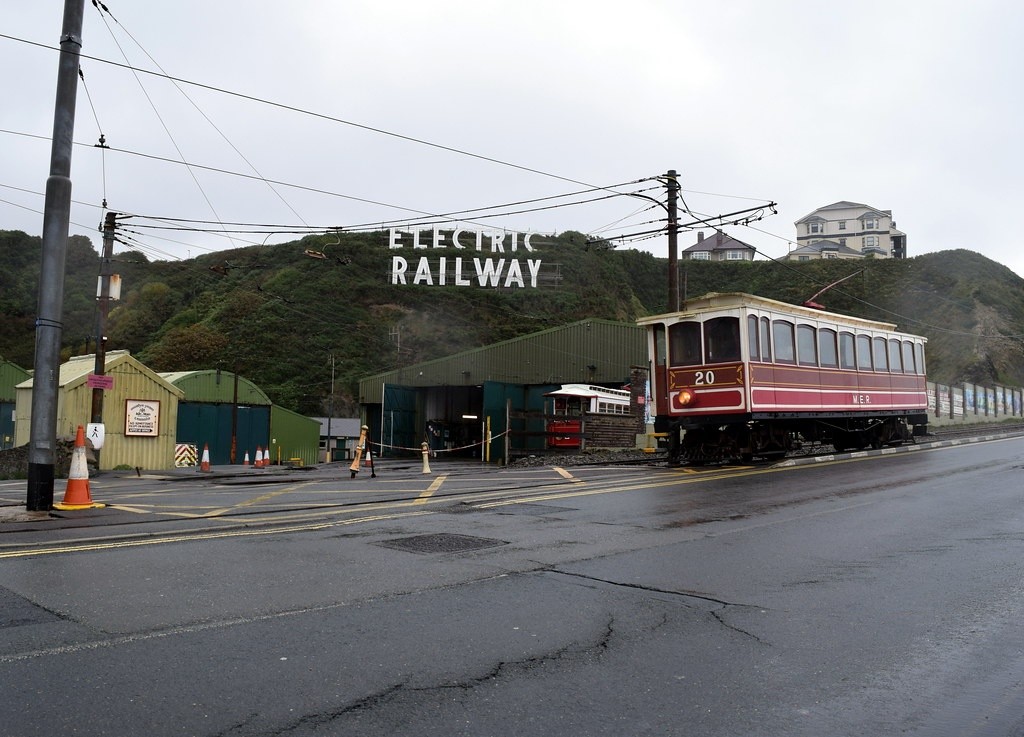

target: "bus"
[634,292,931,467]
[544,383,645,448]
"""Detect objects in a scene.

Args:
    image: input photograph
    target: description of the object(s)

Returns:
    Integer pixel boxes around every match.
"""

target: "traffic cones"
[262,447,272,467]
[250,446,265,470]
[51,425,106,510]
[362,448,375,467]
[196,444,214,473]
[243,449,250,466]
